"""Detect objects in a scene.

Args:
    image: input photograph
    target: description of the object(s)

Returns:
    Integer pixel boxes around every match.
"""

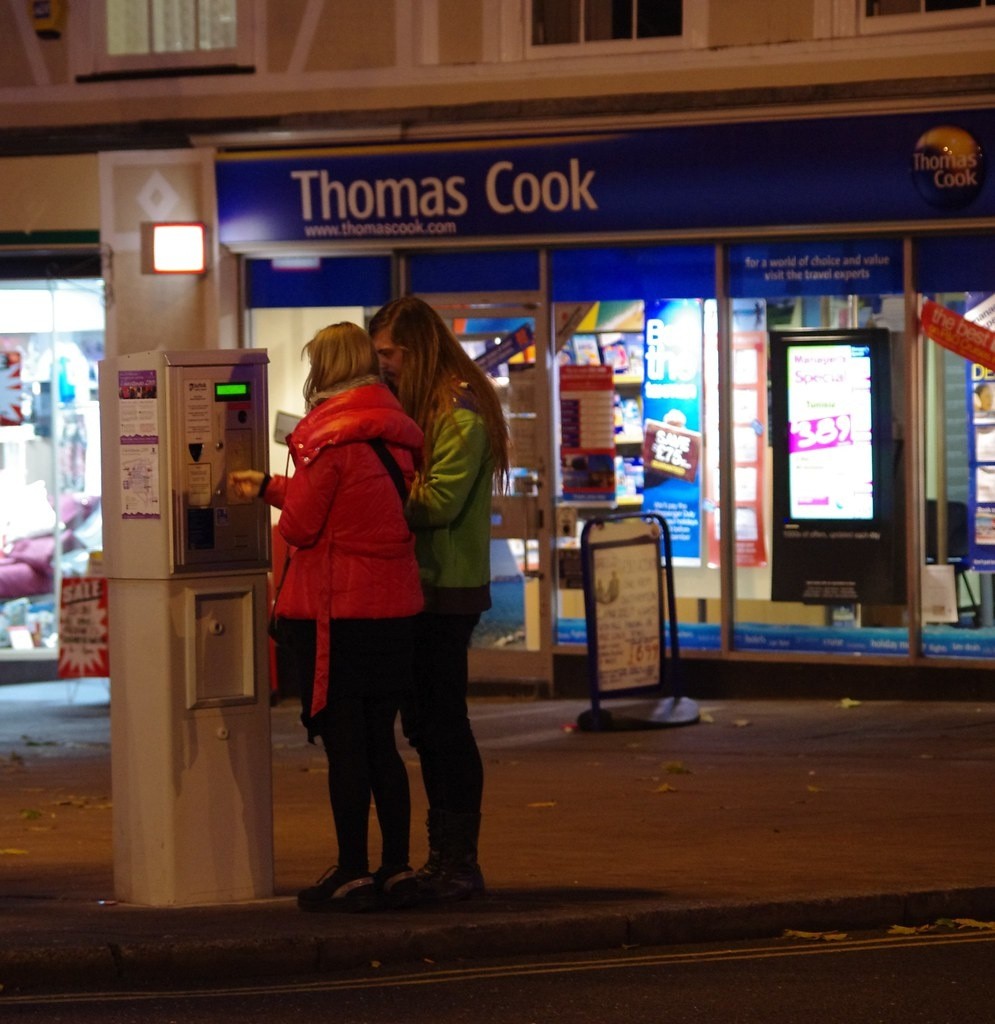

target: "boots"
[413,809,484,903]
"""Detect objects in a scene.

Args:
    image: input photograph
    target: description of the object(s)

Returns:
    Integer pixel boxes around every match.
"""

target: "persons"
[228,322,422,906]
[371,296,511,902]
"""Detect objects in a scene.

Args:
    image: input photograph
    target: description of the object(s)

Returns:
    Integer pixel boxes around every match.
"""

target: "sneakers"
[373,864,419,909]
[297,865,382,913]
